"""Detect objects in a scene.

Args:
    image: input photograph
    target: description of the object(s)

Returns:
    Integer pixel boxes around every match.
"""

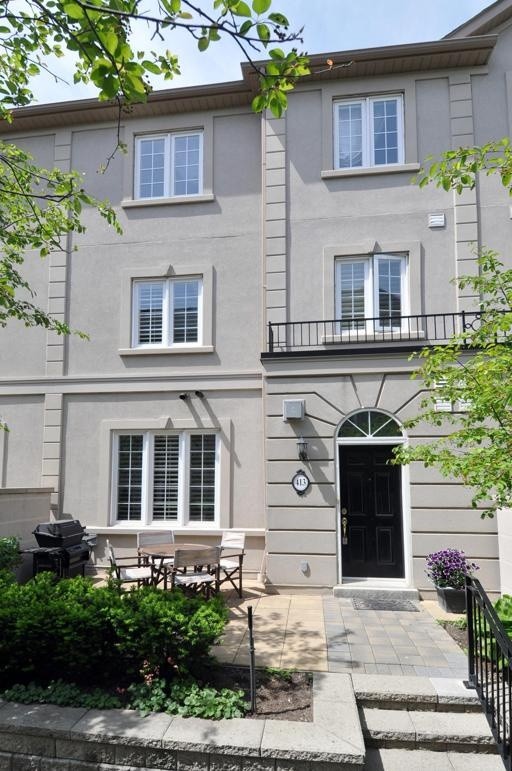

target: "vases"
[433,582,467,612]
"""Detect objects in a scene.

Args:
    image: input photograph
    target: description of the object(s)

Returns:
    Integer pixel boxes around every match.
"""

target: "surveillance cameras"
[180,393,186,399]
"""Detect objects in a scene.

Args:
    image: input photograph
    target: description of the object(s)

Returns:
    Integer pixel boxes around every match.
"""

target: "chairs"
[100,526,248,603]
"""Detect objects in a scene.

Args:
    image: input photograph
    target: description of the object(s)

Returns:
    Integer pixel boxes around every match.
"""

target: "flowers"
[422,545,480,590]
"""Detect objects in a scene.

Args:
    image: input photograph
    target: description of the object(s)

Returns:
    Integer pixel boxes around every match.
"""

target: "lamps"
[296,436,308,461]
